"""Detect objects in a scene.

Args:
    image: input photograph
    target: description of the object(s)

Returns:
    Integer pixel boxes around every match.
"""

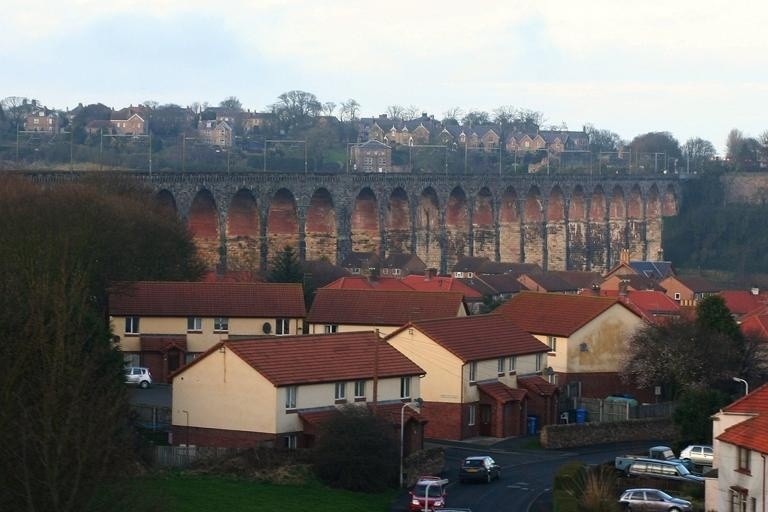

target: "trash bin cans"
[576,409,585,422]
[528,417,537,434]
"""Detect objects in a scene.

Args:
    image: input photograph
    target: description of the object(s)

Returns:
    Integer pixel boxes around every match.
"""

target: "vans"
[123,367,152,389]
[679,445,713,466]
[629,459,704,483]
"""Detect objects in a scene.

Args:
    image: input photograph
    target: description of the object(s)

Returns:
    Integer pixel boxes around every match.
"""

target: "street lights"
[399,401,420,490]
[732,376,748,395]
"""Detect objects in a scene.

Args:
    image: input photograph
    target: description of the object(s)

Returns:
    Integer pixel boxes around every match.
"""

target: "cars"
[460,456,501,483]
[617,488,692,512]
[410,476,448,510]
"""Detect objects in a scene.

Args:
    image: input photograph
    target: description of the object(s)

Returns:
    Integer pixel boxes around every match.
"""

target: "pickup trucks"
[615,446,691,471]
